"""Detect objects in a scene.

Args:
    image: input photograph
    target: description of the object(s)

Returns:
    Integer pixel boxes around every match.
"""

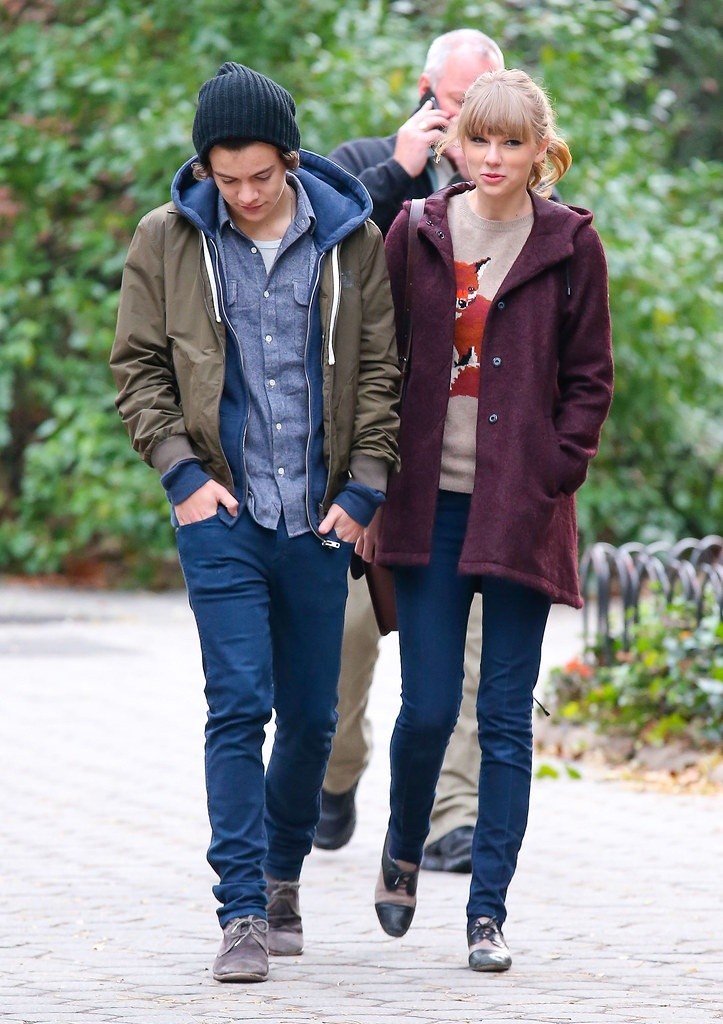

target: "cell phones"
[419,90,444,132]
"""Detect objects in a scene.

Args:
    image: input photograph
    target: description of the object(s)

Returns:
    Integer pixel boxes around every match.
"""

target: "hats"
[193,62,300,170]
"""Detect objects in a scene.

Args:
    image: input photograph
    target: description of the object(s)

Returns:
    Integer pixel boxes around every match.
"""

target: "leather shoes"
[375,827,420,937]
[467,914,512,970]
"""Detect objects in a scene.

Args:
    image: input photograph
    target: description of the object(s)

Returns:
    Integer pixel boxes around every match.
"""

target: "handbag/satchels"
[350,543,398,636]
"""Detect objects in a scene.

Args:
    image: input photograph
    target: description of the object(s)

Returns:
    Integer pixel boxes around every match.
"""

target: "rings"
[418,120,428,132]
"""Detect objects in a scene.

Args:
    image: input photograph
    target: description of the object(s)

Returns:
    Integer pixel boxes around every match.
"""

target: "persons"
[313,29,564,873]
[111,60,404,985]
[349,69,614,972]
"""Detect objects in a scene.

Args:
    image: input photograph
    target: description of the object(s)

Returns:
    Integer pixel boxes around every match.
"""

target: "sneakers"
[421,826,476,873]
[312,765,367,849]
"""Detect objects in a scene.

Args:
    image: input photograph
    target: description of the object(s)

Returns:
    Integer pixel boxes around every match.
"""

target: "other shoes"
[213,914,269,981]
[262,866,304,955]
